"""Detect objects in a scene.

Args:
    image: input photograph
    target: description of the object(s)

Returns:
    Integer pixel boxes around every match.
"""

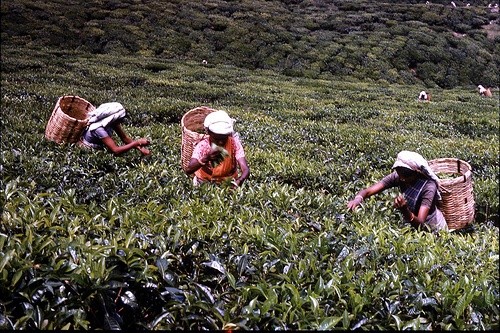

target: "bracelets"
[355,195,363,201]
[238,176,244,182]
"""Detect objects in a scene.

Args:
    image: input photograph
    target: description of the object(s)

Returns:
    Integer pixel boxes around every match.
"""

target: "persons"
[426,0,499,8]
[346,150,448,234]
[477,84,485,95]
[419,91,427,99]
[186,110,248,191]
[202,60,207,64]
[79,102,149,156]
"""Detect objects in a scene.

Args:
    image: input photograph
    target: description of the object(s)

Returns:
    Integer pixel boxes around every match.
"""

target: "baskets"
[426,158,475,230]
[45,96,96,143]
[181,107,217,179]
